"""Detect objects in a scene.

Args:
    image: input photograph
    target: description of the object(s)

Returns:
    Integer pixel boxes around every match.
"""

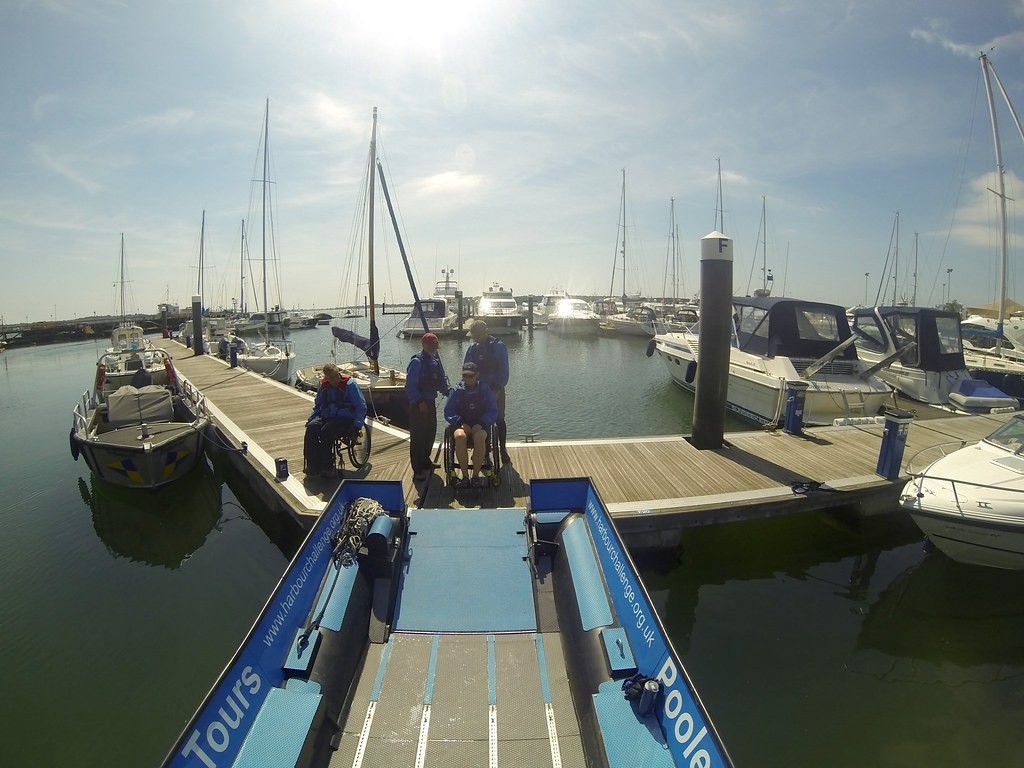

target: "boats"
[546,298,603,334]
[468,284,525,335]
[398,294,458,337]
[70,348,211,491]
[898,411,1024,574]
[430,265,465,316]
[819,302,1022,424]
[522,288,571,321]
[639,296,901,429]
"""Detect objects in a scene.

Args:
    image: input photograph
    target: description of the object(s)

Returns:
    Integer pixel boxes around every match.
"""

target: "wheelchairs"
[442,385,503,491]
[300,413,374,477]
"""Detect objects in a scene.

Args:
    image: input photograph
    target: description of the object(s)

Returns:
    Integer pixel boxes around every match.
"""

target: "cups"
[638,680,659,717]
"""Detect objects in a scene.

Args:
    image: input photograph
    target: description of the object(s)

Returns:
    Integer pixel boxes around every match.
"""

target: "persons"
[443,362,498,489]
[406,333,452,480]
[463,320,511,465]
[303,363,367,477]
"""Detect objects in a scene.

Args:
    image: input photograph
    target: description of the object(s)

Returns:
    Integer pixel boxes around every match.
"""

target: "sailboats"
[292,102,411,430]
[223,97,297,384]
[171,211,291,355]
[894,44,1023,403]
[589,156,953,337]
[93,232,178,405]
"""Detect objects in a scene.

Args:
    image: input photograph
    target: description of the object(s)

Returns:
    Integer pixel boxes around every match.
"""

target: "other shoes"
[426,460,441,468]
[462,478,470,489]
[500,450,510,464]
[481,454,494,470]
[415,467,425,481]
[303,463,319,475]
[471,475,480,488]
[319,463,333,476]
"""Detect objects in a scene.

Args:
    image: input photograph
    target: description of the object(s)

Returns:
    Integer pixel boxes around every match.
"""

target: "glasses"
[463,373,476,378]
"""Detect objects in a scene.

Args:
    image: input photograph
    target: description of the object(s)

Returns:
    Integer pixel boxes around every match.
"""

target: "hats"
[421,332,440,349]
[460,362,477,375]
[466,320,487,337]
